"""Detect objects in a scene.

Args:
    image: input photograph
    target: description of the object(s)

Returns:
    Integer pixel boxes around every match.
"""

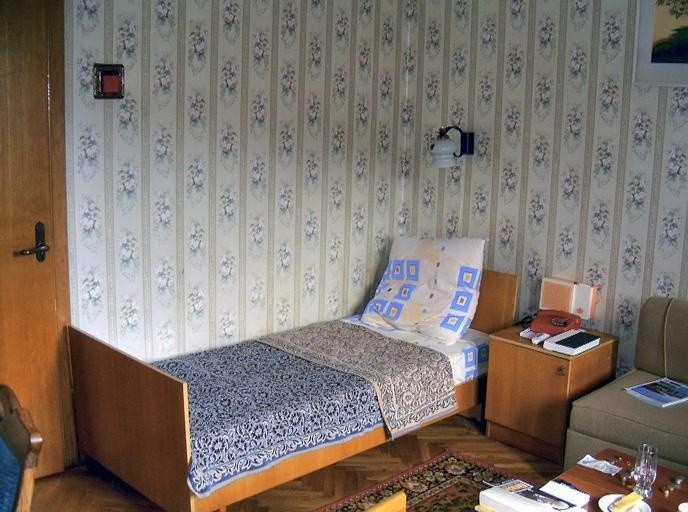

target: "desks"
[539,448,688,512]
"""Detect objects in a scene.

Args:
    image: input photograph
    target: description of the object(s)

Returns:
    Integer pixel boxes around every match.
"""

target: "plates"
[597,493,652,512]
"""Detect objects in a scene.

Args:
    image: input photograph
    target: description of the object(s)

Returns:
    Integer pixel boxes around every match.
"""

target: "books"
[542,326,602,357]
[621,375,688,409]
[474,475,578,511]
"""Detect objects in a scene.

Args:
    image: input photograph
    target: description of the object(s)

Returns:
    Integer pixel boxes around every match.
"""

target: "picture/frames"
[631,0,688,88]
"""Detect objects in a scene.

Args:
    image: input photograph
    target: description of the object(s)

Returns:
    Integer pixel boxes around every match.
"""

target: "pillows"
[359,234,487,347]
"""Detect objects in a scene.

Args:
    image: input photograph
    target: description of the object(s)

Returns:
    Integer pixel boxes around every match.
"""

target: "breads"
[612,491,643,512]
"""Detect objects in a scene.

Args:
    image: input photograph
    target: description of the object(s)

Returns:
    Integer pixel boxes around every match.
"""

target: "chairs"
[561,296,688,475]
[0,383,44,512]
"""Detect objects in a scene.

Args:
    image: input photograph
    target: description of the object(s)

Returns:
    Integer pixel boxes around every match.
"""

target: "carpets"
[309,450,543,512]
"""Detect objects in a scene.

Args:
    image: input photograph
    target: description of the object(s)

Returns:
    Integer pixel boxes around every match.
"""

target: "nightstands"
[485,319,619,467]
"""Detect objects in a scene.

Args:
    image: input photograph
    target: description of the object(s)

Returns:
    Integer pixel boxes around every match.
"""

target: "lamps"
[427,125,476,169]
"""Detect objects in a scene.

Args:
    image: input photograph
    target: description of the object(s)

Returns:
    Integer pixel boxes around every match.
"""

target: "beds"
[67,266,520,512]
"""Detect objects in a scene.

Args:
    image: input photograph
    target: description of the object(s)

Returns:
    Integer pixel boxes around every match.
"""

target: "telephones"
[531,309,581,333]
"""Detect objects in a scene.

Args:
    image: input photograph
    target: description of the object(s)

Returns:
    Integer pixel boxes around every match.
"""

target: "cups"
[634,442,657,500]
[678,502,688,511]
